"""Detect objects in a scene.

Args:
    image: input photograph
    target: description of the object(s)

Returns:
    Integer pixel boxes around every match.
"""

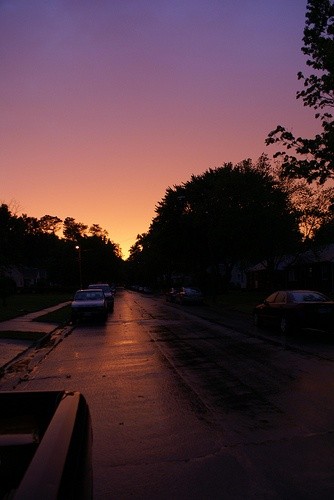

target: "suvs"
[88,283,114,313]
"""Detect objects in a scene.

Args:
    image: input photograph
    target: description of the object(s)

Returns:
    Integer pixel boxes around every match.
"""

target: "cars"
[175,287,204,307]
[165,287,177,303]
[70,289,110,325]
[255,290,334,338]
[110,282,153,296]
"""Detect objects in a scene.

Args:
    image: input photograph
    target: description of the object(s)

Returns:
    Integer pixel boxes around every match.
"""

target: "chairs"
[76,293,101,300]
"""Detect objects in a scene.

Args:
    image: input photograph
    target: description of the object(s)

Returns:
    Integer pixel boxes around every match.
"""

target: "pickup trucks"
[1,388,94,500]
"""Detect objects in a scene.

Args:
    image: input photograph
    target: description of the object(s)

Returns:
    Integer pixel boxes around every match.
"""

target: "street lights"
[75,245,82,290]
